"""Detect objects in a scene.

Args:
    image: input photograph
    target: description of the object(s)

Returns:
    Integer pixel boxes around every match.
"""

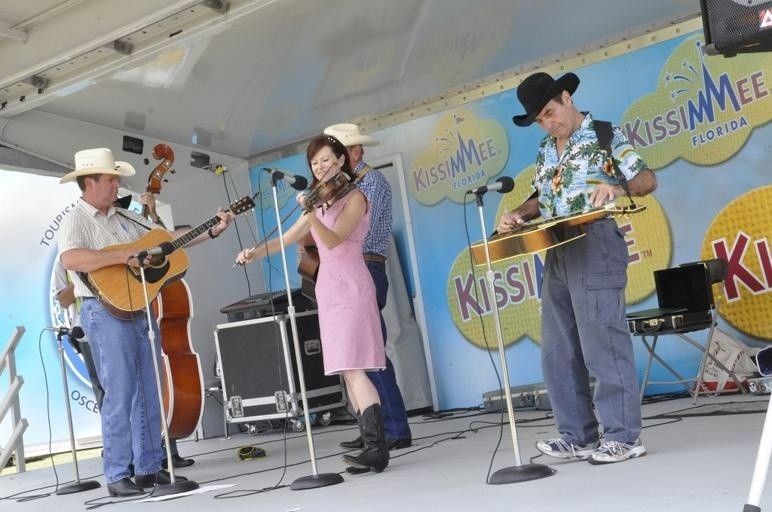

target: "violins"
[305,174,348,213]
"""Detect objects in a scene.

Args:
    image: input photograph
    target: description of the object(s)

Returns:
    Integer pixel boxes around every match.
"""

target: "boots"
[341,402,388,472]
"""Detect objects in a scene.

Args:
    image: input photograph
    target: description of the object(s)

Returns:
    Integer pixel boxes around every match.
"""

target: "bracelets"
[204,230,221,241]
[294,123,412,450]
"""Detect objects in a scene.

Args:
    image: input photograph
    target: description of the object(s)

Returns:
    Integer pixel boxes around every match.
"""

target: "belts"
[363,253,386,263]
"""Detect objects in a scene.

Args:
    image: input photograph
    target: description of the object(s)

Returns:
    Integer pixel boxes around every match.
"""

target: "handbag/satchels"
[693,327,758,394]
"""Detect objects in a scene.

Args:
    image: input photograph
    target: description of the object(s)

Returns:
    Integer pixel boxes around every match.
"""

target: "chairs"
[625,257,748,408]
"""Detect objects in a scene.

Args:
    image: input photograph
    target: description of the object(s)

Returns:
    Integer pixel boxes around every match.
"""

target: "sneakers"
[536,439,601,460]
[588,436,645,465]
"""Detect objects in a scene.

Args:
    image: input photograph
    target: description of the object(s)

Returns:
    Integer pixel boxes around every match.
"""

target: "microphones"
[139,242,174,256]
[466,177,514,194]
[270,168,307,190]
[54,327,84,338]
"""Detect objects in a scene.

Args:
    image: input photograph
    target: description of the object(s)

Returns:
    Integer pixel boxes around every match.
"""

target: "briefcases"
[624,263,715,335]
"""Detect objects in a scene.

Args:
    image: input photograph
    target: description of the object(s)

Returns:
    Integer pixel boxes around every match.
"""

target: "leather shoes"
[135,471,186,489]
[387,438,412,450]
[107,478,145,498]
[161,454,195,468]
[339,436,363,449]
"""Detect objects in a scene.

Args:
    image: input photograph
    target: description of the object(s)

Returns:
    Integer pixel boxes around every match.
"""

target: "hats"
[116,195,132,209]
[324,123,380,147]
[59,148,135,184]
[513,72,580,127]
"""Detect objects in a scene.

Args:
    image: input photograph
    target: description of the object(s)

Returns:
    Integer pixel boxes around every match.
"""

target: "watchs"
[153,216,163,225]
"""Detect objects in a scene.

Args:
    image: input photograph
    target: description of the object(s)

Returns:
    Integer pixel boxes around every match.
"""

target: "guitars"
[298,246,320,302]
[87,196,255,321]
[471,205,645,266]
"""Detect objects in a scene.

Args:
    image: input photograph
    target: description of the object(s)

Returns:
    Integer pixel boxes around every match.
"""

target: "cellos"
[140,145,205,440]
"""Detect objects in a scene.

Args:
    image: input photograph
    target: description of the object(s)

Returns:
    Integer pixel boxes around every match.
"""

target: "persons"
[234,134,389,473]
[51,260,195,468]
[496,71,657,465]
[56,146,234,499]
